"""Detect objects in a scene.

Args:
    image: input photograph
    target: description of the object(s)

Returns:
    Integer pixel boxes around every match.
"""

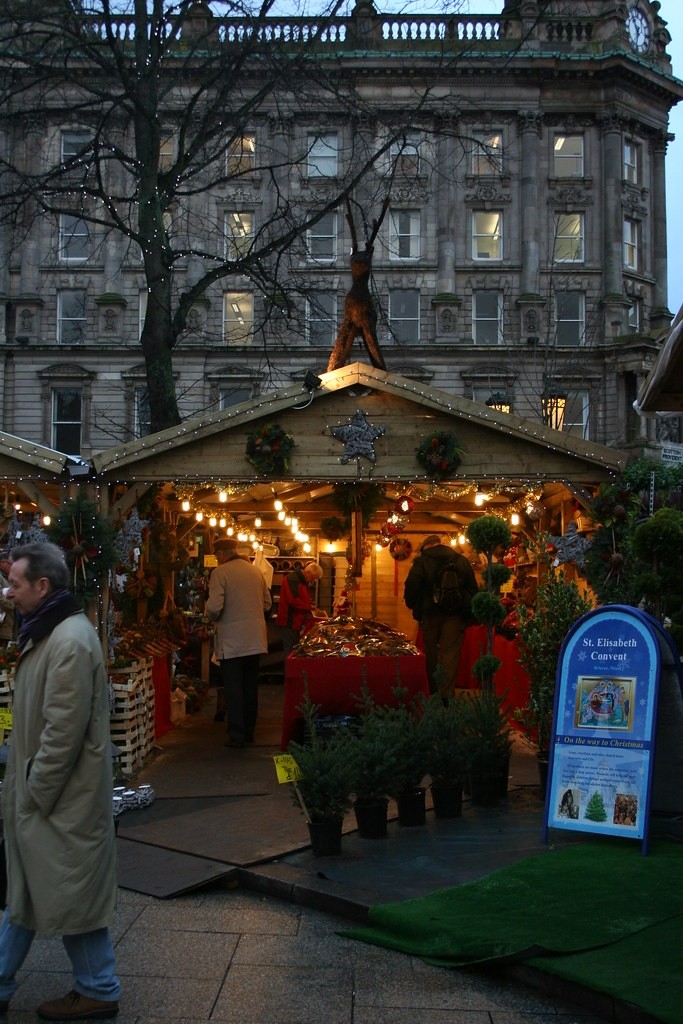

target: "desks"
[281,648,430,747]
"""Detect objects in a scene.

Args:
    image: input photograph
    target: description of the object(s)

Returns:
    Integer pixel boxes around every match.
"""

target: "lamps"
[15,337,30,345]
[527,337,539,344]
[67,466,92,479]
[303,369,322,389]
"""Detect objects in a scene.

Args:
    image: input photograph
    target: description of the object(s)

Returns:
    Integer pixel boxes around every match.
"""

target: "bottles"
[122,791,139,810]
[112,797,123,816]
[137,785,155,806]
[113,786,126,798]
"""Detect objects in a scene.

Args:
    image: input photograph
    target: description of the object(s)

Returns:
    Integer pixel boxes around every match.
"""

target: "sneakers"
[37,989,119,1021]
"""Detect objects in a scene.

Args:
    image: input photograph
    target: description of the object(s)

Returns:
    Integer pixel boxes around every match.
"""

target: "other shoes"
[245,737,254,745]
[224,735,243,748]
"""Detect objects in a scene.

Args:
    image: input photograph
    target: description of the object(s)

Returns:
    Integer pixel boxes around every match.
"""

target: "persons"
[277,562,324,656]
[0,539,121,1019]
[404,534,477,707]
[204,538,272,748]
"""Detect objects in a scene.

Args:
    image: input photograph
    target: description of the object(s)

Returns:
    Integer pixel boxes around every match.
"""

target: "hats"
[214,538,239,552]
[419,536,440,551]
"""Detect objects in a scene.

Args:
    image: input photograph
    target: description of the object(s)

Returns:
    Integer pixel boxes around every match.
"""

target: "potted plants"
[275,656,516,855]
[517,529,592,793]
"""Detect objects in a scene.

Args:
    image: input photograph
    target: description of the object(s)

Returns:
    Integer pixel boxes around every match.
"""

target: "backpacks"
[427,553,467,617]
